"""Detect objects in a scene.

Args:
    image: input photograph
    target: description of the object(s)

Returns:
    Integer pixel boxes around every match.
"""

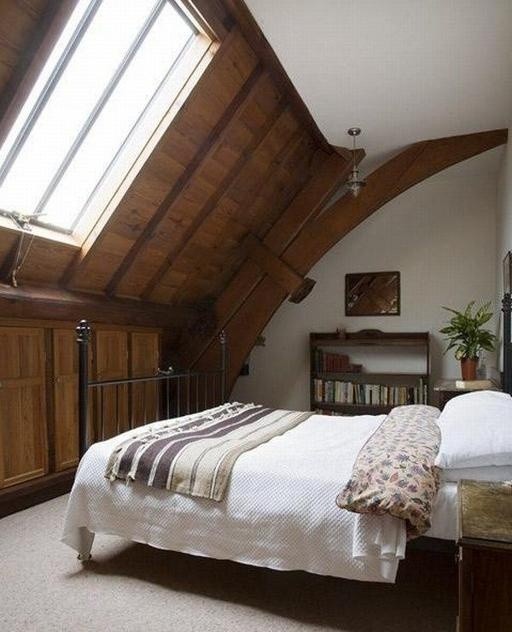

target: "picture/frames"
[345,271,400,317]
[502,251,512,294]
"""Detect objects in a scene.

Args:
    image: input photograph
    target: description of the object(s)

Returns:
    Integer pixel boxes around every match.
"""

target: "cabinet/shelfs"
[309,332,431,416]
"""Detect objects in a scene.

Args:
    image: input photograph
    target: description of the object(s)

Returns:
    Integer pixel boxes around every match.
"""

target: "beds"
[60,294,511,600]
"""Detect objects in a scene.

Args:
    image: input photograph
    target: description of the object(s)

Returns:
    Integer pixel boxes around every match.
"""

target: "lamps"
[346,127,366,197]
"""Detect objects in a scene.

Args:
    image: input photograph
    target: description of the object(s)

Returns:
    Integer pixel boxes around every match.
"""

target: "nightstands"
[434,380,500,410]
[456,480,512,632]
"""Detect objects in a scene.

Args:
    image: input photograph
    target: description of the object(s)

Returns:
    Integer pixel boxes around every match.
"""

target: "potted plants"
[439,300,497,381]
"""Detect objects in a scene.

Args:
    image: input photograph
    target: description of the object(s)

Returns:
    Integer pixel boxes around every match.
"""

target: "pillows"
[434,389,512,469]
[438,464,511,483]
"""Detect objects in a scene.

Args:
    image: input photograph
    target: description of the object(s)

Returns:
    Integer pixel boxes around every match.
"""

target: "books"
[314,377,427,407]
[313,347,361,375]
